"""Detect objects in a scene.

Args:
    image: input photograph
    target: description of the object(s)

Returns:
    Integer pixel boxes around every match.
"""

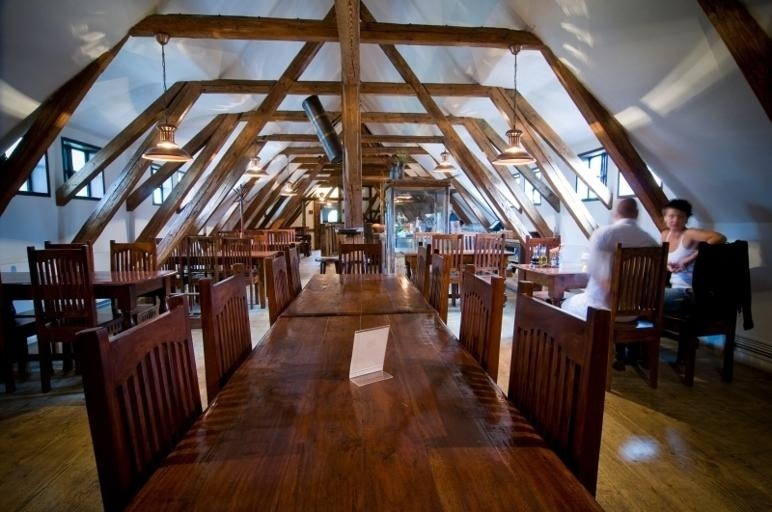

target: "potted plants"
[391,150,412,179]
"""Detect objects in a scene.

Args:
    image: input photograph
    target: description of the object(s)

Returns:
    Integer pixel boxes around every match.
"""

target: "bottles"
[531,246,561,267]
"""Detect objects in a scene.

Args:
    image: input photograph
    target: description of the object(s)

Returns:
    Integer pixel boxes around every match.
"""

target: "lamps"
[279,154,297,197]
[246,143,268,177]
[434,144,458,174]
[491,45,537,167]
[143,32,195,164]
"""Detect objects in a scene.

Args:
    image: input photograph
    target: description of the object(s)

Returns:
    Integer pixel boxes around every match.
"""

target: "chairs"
[74,294,201,511]
[509,280,612,504]
[1,223,771,392]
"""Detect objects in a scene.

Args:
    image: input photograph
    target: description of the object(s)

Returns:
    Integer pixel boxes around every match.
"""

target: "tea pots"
[389,161,404,179]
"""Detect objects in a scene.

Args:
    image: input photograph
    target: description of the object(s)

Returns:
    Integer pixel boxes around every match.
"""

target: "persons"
[625,200,726,366]
[560,199,660,372]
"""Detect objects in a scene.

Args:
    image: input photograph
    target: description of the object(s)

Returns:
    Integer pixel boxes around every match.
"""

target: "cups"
[581,245,591,267]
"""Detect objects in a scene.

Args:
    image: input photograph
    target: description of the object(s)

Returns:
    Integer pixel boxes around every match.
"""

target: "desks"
[132,312,604,512]
